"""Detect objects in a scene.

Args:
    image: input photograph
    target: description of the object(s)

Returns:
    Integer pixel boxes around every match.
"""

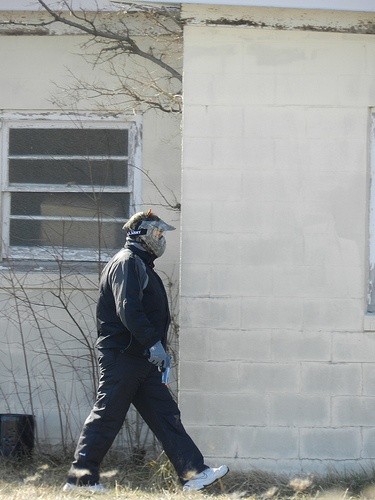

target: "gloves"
[148,340,167,368]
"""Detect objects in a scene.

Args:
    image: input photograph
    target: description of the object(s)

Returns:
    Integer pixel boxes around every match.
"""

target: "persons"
[61,212,228,493]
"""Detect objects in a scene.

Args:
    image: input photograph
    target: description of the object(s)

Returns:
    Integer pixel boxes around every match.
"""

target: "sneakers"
[62,482,106,494]
[183,464,229,494]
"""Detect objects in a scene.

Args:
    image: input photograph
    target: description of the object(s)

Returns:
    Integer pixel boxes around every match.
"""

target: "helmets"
[123,211,177,257]
